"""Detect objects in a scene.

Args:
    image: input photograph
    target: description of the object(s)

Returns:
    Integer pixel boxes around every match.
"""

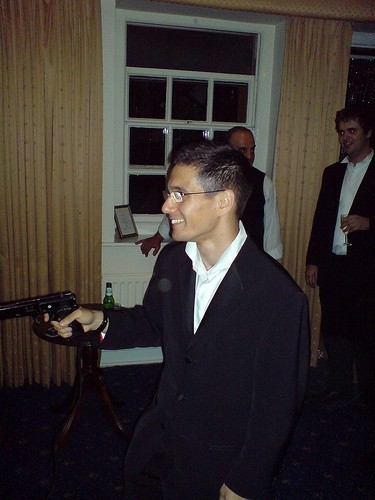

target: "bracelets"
[95,310,108,334]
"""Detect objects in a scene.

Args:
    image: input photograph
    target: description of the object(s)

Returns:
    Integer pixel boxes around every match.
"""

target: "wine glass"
[341,214,352,246]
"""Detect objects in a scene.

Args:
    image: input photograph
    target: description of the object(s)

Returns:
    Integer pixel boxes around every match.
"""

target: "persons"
[304,107,375,407]
[133,126,285,269]
[43,139,314,500]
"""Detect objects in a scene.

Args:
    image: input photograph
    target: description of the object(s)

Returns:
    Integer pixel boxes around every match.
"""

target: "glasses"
[162,189,226,203]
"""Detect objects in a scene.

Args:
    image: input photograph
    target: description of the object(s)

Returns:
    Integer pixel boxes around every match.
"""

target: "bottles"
[103,282,114,310]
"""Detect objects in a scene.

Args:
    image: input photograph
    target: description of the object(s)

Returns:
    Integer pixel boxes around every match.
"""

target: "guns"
[1,290,84,340]
